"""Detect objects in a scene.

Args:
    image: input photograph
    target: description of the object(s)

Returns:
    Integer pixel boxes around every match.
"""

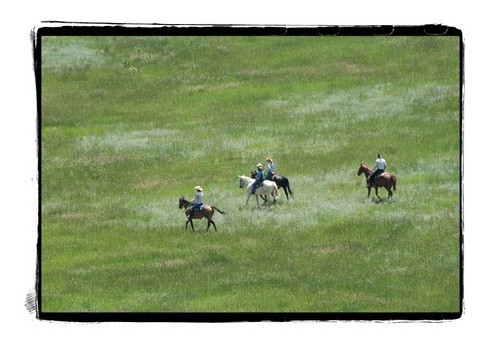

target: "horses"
[238,174,280,211]
[357,163,397,203]
[250,170,295,202]
[179,196,226,233]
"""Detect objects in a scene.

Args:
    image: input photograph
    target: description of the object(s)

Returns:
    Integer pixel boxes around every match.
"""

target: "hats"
[195,186,203,191]
[266,157,272,162]
[257,163,263,169]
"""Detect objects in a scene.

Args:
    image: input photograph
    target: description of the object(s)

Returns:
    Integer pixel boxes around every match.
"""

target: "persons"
[251,162,265,194]
[368,154,387,188]
[188,185,203,219]
[265,157,276,178]
